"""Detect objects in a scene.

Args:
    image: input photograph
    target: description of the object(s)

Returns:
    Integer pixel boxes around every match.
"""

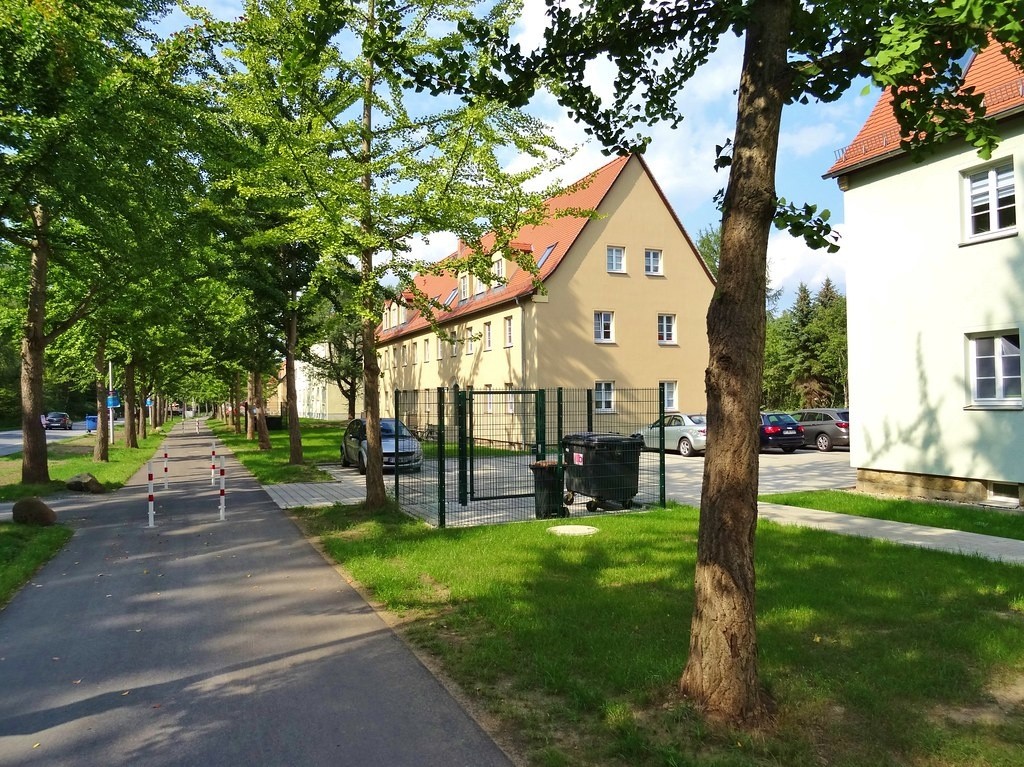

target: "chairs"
[382,422,393,433]
[808,415,816,421]
[674,419,682,425]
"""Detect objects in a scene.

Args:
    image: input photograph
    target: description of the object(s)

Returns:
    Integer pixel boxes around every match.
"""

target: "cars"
[790,408,849,451]
[635,414,708,456]
[46,412,73,430]
[760,413,806,454]
[226,408,236,416]
[107,409,118,420]
[339,417,422,475]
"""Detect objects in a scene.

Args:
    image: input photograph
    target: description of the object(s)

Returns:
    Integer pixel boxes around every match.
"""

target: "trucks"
[164,404,182,416]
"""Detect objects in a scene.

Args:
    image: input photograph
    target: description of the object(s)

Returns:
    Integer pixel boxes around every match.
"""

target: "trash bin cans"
[85,416,98,433]
[561,431,645,512]
[529,460,570,520]
